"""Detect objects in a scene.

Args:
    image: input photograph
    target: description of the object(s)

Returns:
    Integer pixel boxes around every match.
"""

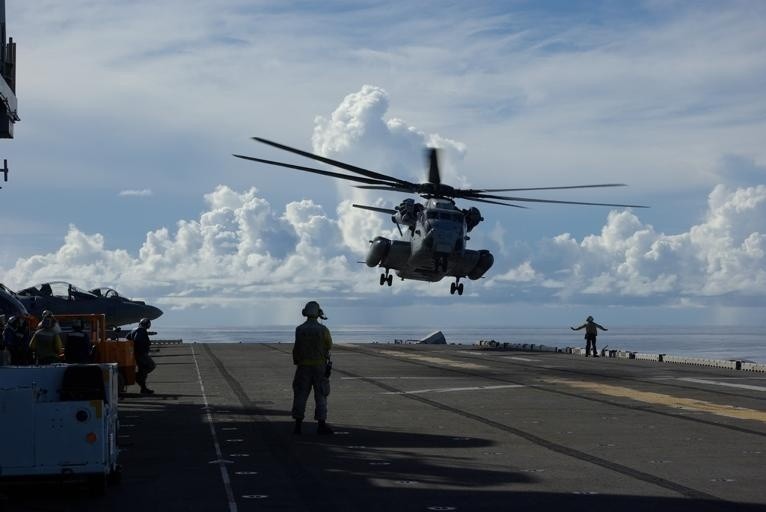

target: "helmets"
[139,318,150,329]
[7,310,56,330]
[586,316,593,321]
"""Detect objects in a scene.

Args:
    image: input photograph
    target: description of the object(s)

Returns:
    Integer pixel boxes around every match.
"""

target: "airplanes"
[0,281,165,339]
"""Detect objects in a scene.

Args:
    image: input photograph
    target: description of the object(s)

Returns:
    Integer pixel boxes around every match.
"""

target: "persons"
[570,315,608,357]
[0,310,92,364]
[289,300,334,437]
[125,317,156,394]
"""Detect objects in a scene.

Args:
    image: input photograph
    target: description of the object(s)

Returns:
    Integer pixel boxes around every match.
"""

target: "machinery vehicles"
[0,364,122,481]
[24,314,137,395]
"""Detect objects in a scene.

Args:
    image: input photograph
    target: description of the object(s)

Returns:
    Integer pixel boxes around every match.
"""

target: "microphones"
[320,316,328,320]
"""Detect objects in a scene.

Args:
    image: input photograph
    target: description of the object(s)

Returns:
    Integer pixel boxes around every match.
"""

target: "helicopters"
[230,138,652,295]
